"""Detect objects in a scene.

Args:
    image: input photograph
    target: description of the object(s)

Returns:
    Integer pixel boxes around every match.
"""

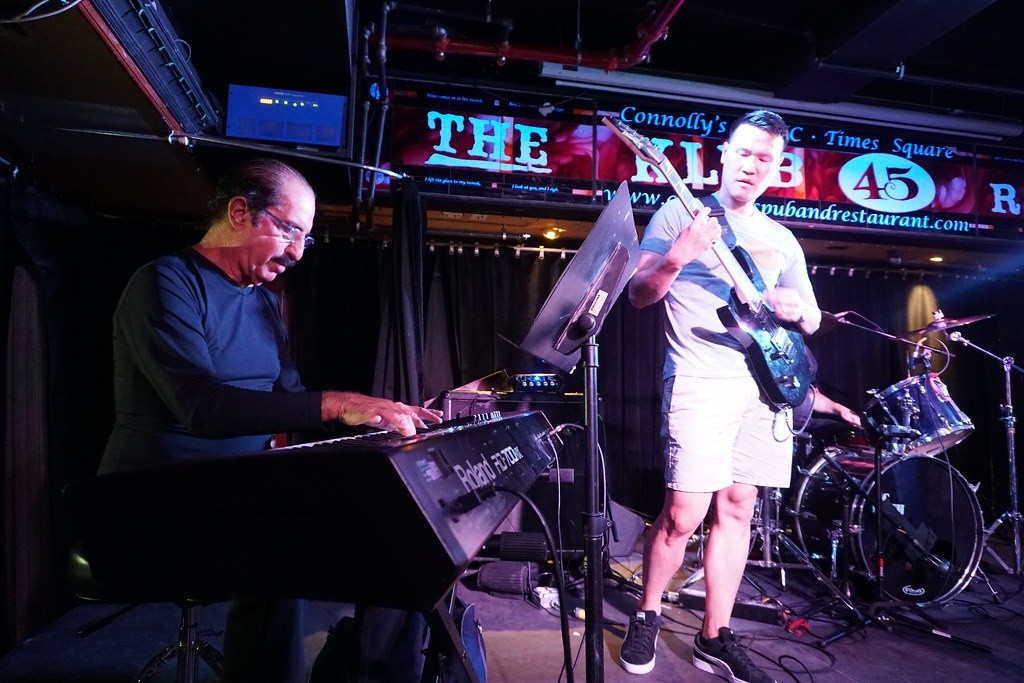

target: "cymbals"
[896,313,996,337]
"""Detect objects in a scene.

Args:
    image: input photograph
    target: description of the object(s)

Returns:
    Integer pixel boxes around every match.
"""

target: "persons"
[618,110,821,683]
[791,345,863,465]
[98,153,445,683]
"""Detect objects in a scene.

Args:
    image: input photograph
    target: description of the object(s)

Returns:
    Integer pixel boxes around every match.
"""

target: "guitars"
[601,115,818,410]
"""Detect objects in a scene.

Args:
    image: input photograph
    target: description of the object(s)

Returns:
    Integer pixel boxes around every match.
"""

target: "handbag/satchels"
[310,594,487,683]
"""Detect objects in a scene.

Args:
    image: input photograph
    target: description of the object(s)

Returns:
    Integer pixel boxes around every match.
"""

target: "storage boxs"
[441,388,607,555]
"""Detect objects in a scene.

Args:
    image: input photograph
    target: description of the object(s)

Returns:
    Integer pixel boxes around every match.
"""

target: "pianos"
[31,410,568,615]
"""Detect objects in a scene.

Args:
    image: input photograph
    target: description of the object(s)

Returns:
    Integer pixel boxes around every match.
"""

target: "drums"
[791,445,988,609]
[863,370,977,458]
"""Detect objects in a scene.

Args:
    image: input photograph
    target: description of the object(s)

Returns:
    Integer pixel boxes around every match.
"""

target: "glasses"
[245,195,316,250]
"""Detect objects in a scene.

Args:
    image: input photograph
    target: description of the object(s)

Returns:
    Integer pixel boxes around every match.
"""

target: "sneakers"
[692,626,777,683]
[619,607,662,674]
